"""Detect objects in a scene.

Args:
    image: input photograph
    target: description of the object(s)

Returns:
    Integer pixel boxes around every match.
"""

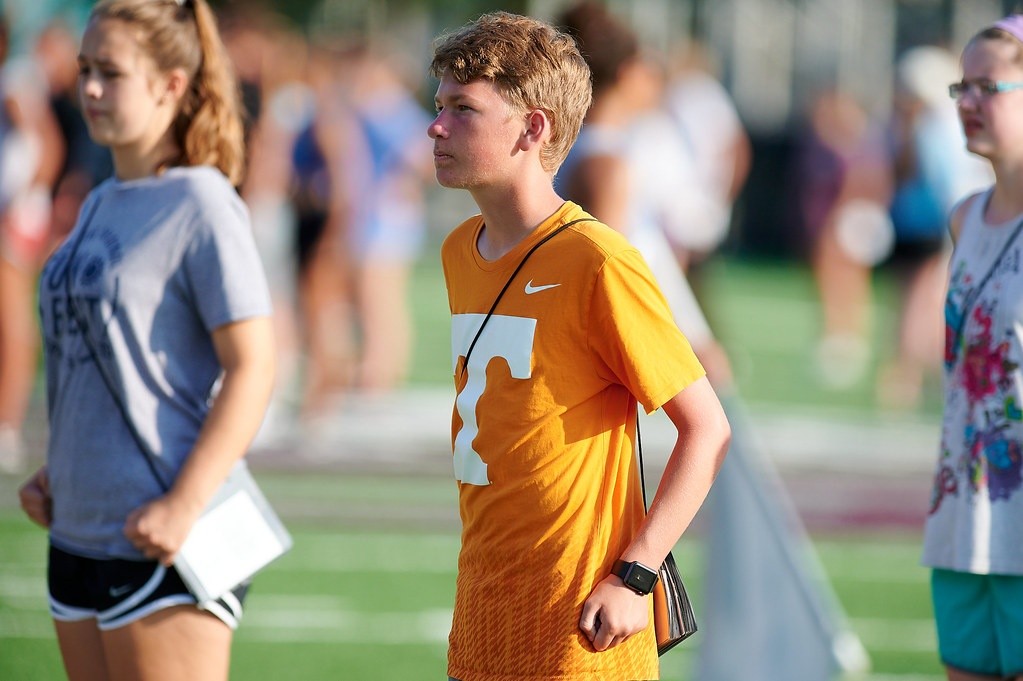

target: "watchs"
[610,559,660,596]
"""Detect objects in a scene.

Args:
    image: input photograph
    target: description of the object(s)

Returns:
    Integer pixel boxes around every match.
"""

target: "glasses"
[949,80,1023,100]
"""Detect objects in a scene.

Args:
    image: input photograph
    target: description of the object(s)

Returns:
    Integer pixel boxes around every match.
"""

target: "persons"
[15,0,279,681]
[556,6,753,383]
[426,11,732,681]
[0,0,437,476]
[923,14,1023,681]
[798,47,981,386]
